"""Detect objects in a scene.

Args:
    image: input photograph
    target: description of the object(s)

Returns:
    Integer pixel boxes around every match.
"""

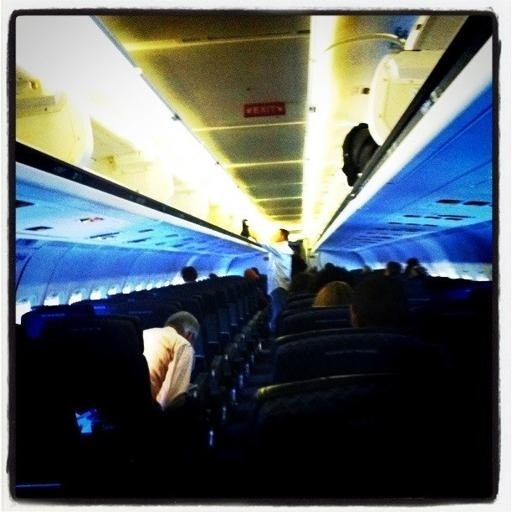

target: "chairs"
[11,271,498,505]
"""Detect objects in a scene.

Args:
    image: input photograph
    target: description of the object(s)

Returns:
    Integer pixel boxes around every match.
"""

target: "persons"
[141,311,204,409]
[180,264,199,283]
[288,254,429,311]
[349,275,410,332]
[244,267,257,280]
[252,226,296,335]
